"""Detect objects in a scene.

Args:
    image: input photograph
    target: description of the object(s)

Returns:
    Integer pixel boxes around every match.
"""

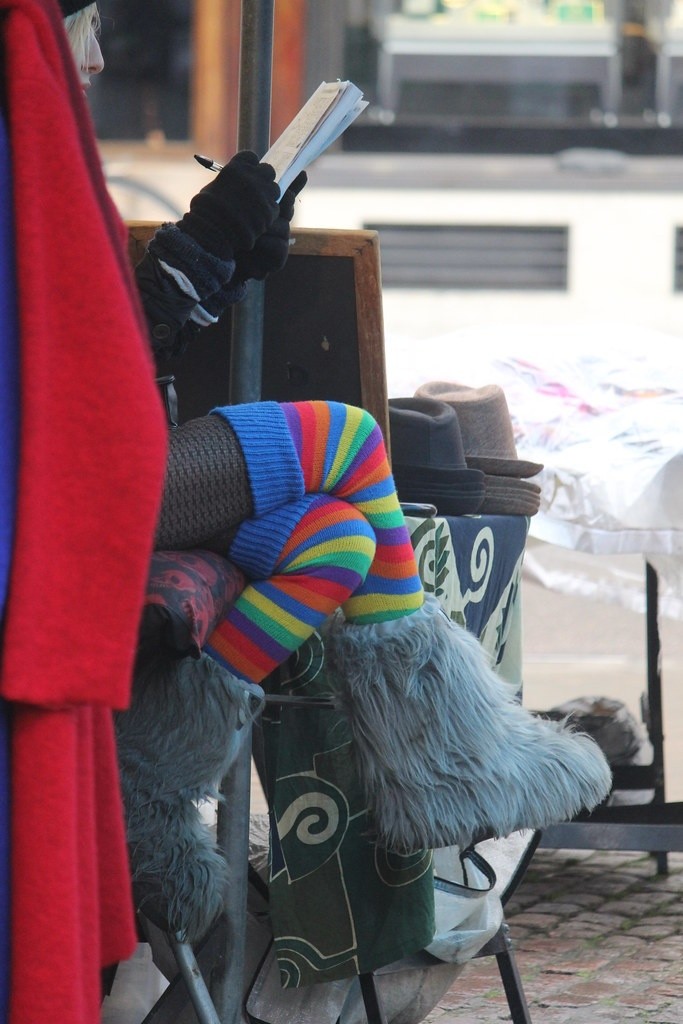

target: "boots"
[319,590,640,850]
[111,649,268,942]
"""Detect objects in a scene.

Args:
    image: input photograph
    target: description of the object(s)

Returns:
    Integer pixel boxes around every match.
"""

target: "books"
[256,78,370,203]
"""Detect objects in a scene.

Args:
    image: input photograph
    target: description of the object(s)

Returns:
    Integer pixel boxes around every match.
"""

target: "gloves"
[198,169,308,318]
[145,149,282,301]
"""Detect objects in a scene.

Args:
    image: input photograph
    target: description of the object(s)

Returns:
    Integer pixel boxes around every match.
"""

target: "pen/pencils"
[192,151,224,174]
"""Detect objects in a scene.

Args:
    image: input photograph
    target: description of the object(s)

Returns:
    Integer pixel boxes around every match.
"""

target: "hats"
[414,380,544,516]
[388,397,486,515]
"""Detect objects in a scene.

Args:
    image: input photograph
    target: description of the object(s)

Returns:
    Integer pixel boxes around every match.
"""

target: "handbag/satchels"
[422,826,538,967]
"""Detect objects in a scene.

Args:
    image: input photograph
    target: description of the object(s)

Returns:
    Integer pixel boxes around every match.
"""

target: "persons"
[52,1,620,935]
